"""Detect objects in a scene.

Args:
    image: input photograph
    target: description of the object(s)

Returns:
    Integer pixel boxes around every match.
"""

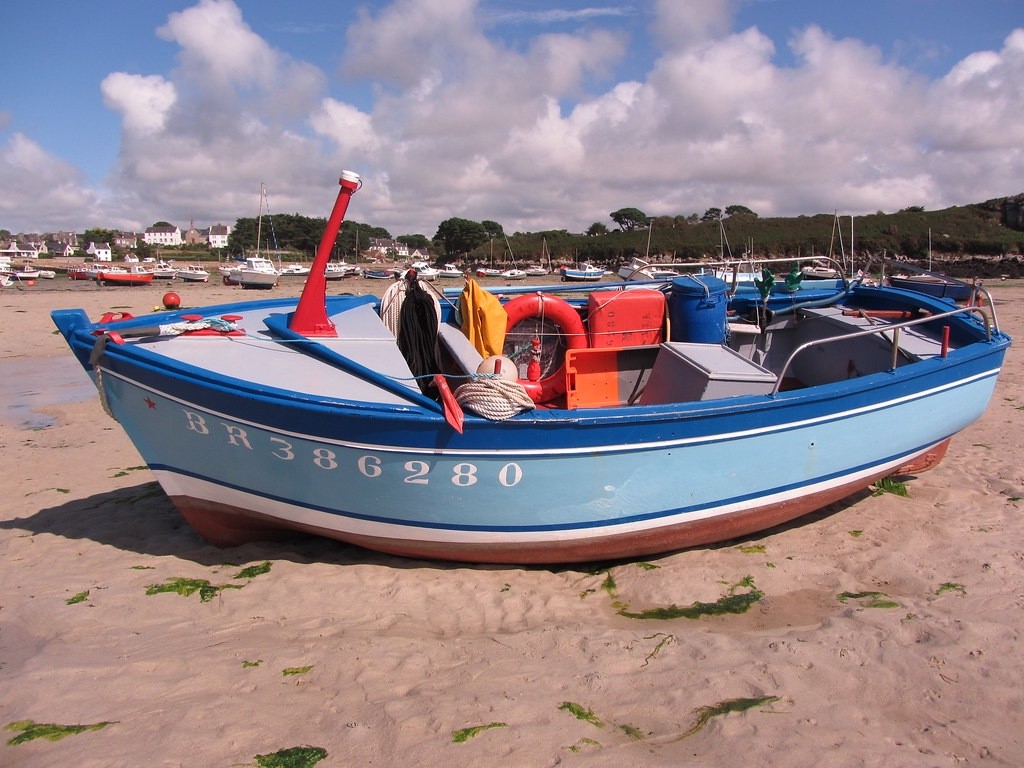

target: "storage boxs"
[639,341,780,409]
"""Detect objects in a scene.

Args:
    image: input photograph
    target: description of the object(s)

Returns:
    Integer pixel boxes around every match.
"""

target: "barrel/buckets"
[668,276,728,344]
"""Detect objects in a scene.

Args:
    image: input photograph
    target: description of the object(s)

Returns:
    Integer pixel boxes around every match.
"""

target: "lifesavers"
[504,294,590,403]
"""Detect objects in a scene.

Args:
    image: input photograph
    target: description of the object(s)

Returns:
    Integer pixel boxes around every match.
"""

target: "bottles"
[528,340,542,382]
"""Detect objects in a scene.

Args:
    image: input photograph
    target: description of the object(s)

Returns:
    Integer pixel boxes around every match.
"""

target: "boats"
[49,171,1015,567]
[0,182,985,319]
